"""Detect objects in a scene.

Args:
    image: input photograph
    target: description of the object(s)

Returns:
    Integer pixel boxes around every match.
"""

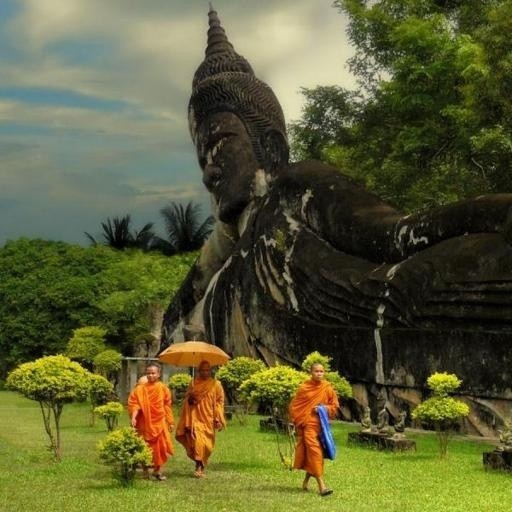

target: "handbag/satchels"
[318,406,336,460]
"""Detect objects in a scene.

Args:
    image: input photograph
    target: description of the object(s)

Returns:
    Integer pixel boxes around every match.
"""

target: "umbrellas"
[159,337,231,389]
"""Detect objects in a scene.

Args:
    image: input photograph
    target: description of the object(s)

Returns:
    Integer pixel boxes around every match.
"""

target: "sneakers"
[194,470,201,477]
[150,469,166,481]
[320,488,333,496]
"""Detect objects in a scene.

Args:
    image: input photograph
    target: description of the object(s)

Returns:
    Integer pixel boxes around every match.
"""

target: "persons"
[289,362,340,497]
[128,363,174,481]
[174,360,225,477]
[155,1,512,412]
[359,398,408,438]
